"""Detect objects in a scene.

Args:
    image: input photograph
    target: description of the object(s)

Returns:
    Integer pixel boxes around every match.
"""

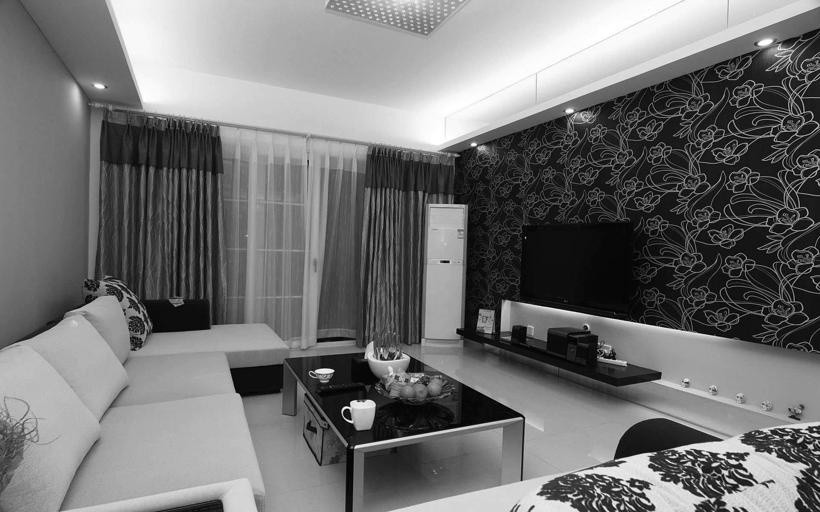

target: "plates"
[373,373,455,406]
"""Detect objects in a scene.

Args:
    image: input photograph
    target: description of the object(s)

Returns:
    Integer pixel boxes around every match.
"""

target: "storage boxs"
[302,393,380,467]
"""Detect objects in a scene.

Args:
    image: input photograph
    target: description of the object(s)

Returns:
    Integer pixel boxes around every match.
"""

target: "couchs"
[0,315,266,512]
[392,417,724,512]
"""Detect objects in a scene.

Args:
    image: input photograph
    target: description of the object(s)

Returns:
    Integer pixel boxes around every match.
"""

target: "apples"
[399,386,414,398]
[414,384,428,399]
[428,380,441,397]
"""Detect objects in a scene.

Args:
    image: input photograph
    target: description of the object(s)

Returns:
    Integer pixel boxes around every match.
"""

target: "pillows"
[511,424,820,512]
[0,274,154,512]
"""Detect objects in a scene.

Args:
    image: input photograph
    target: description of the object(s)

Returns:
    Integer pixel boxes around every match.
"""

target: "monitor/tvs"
[519,221,632,318]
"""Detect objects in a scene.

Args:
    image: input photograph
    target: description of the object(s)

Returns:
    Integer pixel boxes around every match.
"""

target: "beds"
[69,294,290,397]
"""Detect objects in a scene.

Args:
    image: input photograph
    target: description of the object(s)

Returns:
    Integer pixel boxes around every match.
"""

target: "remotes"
[317,380,362,395]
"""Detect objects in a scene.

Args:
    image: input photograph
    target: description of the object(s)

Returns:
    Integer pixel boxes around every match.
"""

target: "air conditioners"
[422,202,470,349]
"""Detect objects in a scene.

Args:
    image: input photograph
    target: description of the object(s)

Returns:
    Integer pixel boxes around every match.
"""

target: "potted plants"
[367,320,411,378]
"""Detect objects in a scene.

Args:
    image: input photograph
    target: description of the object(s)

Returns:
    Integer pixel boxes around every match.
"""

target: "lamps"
[323,0,472,39]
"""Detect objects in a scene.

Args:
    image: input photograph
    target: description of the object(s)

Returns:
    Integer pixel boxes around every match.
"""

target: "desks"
[456,326,662,388]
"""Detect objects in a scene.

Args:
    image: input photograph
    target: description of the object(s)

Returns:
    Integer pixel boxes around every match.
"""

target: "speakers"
[545,325,590,359]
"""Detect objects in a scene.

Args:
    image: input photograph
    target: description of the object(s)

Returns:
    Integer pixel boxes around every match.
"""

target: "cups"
[340,398,376,432]
[308,368,335,384]
